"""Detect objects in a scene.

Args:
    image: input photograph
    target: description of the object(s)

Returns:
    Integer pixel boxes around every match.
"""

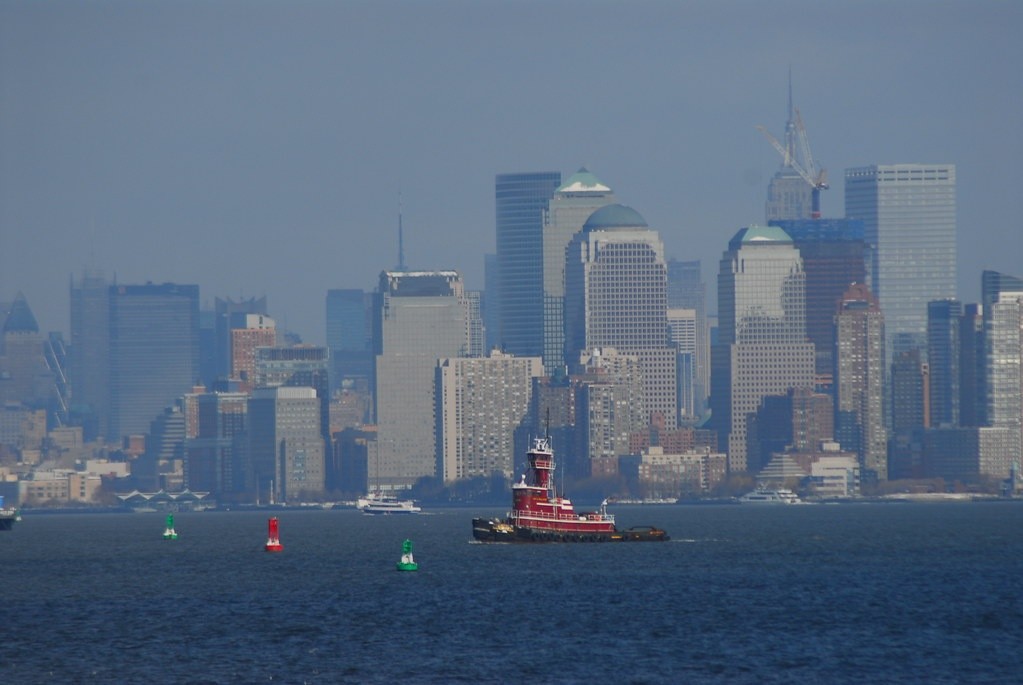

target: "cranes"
[755,109,830,218]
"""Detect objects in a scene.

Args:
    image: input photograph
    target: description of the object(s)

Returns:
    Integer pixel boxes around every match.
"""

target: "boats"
[162,514,178,540]
[266,516,283,551]
[357,487,421,515]
[472,407,671,543]
[0,504,22,530]
[608,496,679,504]
[396,538,418,571]
[738,481,801,505]
